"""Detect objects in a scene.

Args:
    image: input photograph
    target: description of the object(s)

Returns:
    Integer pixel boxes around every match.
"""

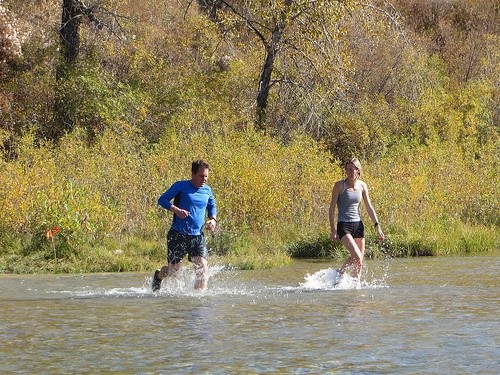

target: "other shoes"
[152,269,163,292]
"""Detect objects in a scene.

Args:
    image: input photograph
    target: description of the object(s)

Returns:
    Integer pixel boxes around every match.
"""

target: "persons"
[151,159,218,293]
[328,157,385,287]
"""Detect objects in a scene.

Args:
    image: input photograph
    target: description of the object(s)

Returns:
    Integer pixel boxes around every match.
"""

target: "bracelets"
[212,218,217,222]
[375,222,379,227]
